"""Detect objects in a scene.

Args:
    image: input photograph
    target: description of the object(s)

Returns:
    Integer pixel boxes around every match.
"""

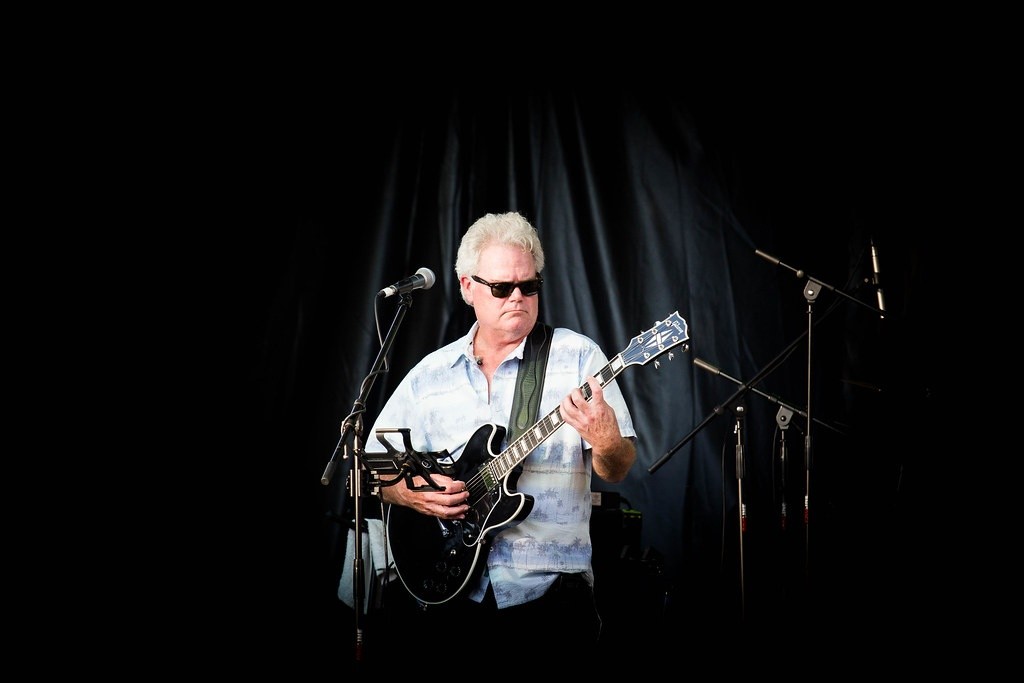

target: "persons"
[363,211,636,683]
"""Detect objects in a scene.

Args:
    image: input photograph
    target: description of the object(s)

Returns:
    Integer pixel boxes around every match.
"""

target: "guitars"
[386,310,690,613]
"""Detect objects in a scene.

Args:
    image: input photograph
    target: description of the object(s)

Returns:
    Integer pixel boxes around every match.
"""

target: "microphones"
[870,245,885,318]
[376,267,436,297]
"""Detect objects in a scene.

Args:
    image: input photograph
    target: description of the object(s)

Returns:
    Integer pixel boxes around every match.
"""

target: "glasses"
[471,272,544,299]
